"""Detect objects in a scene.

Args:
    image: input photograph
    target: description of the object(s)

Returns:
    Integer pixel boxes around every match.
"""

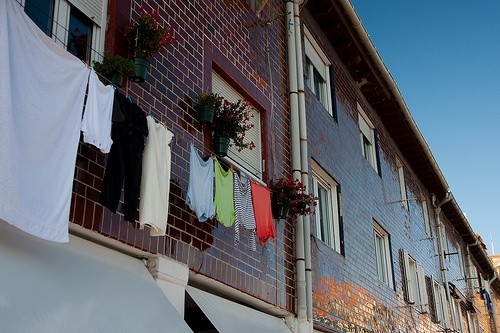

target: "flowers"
[210,96,256,152]
[267,177,318,216]
[123,13,180,57]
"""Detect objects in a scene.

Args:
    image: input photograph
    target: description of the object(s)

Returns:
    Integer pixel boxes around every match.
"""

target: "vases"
[128,57,150,83]
[273,201,288,219]
[213,137,230,156]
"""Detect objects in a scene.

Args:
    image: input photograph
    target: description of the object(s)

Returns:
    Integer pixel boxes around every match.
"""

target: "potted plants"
[94,52,136,87]
[198,90,223,125]
